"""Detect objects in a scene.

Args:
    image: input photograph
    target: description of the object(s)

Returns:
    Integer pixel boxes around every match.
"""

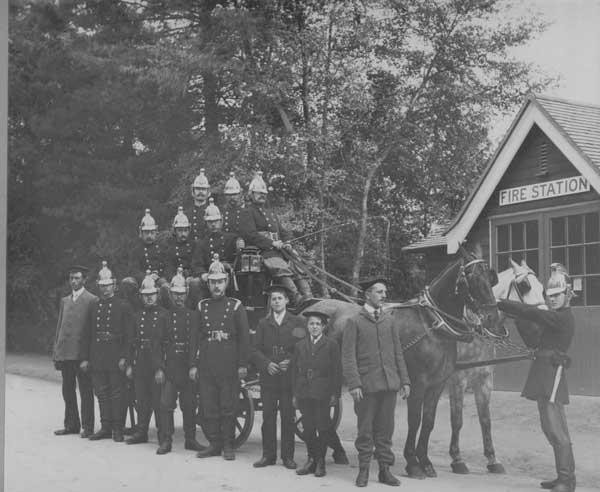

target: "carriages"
[181,211,545,479]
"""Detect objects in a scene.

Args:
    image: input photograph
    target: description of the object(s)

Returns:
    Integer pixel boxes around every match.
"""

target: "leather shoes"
[196,441,223,458]
[314,457,326,477]
[154,438,176,456]
[294,292,314,310]
[124,431,149,444]
[223,441,236,461]
[355,461,370,488]
[295,457,315,476]
[252,453,278,467]
[540,477,578,492]
[184,438,207,452]
[112,424,124,442]
[281,456,298,470]
[53,428,79,436]
[81,425,111,440]
[377,466,402,487]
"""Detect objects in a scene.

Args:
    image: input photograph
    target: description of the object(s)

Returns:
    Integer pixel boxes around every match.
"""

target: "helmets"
[94,260,116,285]
[247,170,269,196]
[205,253,229,280]
[167,267,190,294]
[138,268,159,295]
[542,260,575,296]
[223,171,243,195]
[171,206,192,229]
[202,196,223,222]
[138,208,158,231]
[190,167,210,190]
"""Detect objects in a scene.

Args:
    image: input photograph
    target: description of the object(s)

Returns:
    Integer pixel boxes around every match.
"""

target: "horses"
[295,240,499,480]
[444,259,549,476]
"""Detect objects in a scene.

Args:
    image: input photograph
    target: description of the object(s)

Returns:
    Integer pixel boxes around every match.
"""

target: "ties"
[373,309,379,321]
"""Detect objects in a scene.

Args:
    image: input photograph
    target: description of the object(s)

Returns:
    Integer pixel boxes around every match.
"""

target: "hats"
[66,265,90,275]
[301,310,331,322]
[262,284,294,296]
[357,277,390,292]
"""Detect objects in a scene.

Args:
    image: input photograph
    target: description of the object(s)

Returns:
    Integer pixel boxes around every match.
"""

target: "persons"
[495,263,576,490]
[288,311,343,478]
[340,274,412,488]
[53,169,313,468]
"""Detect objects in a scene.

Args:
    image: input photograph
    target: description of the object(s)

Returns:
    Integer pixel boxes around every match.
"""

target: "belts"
[297,367,331,379]
[197,331,238,342]
[164,342,194,353]
[259,231,279,242]
